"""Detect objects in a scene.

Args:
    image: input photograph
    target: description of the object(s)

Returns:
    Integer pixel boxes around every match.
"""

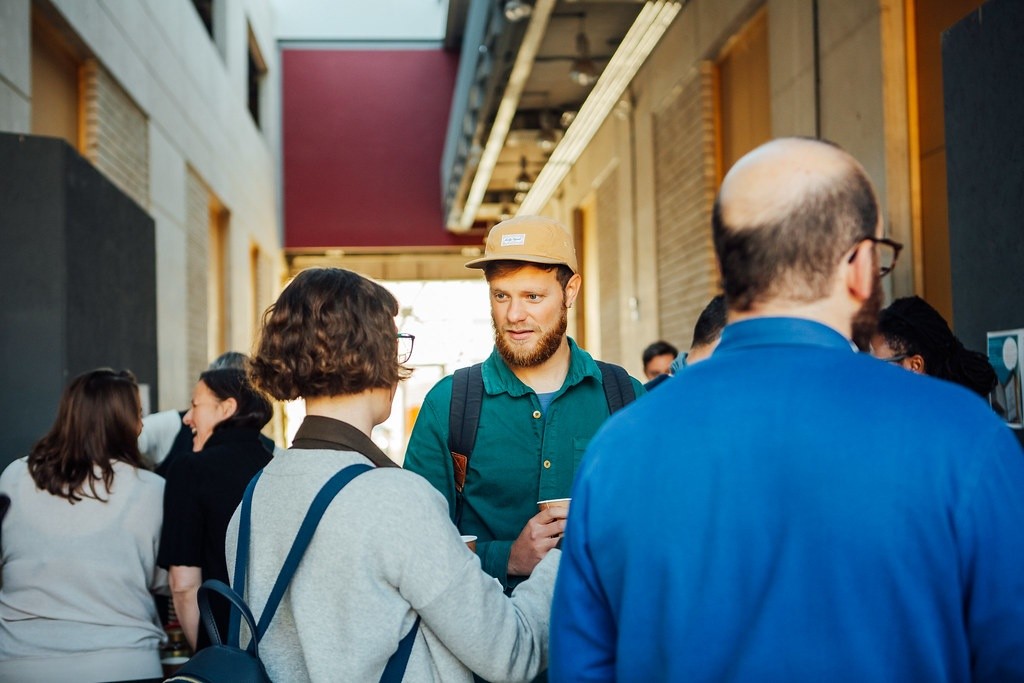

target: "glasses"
[396,333,415,364]
[847,236,903,278]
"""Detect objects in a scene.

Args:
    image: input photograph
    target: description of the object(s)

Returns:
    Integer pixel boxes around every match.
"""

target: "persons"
[550,135,1024,683]
[0,368,170,683]
[869,294,1000,397]
[137,350,285,652]
[643,339,678,384]
[401,216,654,595]
[641,294,728,392]
[225,266,566,683]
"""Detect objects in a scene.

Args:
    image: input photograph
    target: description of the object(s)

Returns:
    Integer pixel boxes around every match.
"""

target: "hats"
[465,215,578,275]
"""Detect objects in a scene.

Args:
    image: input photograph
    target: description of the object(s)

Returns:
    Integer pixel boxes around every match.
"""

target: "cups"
[537,498,571,538]
[461,535,477,554]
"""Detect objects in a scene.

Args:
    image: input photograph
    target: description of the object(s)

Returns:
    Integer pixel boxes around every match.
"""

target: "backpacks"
[159,464,419,683]
